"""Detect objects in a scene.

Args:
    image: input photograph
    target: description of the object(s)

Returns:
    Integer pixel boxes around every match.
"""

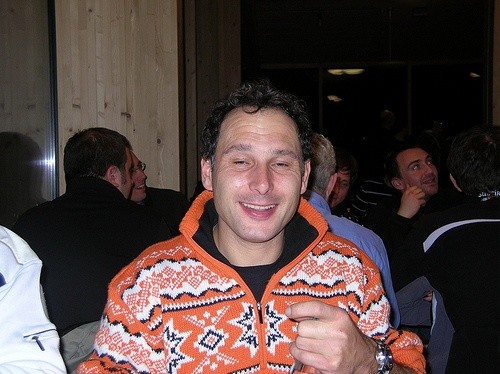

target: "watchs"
[368,337,394,373]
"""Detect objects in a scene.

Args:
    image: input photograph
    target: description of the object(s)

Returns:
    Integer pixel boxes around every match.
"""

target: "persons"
[75,80,429,374]
[10,126,176,374]
[327,144,365,228]
[299,130,402,329]
[362,137,459,262]
[389,123,500,374]
[0,223,69,373]
[128,148,192,238]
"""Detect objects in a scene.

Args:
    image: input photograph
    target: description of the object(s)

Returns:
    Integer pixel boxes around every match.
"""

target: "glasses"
[133,162,146,174]
